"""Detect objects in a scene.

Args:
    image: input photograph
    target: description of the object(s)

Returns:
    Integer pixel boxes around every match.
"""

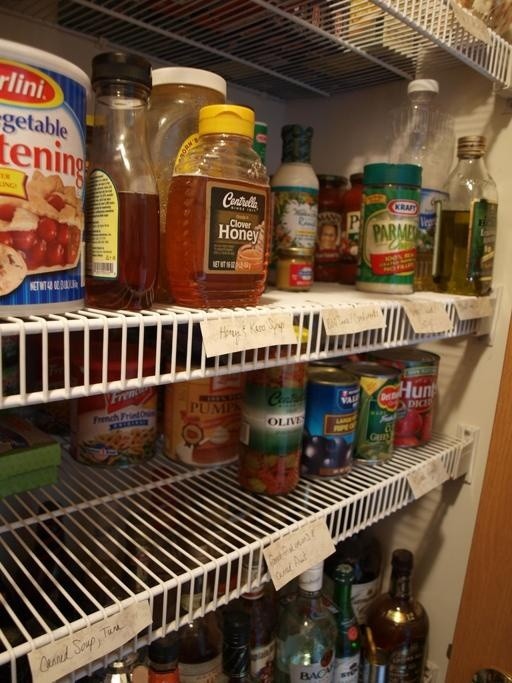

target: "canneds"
[70,343,163,467]
[239,326,310,495]
[304,366,360,477]
[340,173,366,283]
[380,349,442,449]
[0,40,87,317]
[343,361,402,464]
[165,362,242,469]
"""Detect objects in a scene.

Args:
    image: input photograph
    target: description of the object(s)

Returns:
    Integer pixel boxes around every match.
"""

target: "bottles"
[342,174,363,287]
[440,135,498,297]
[277,560,336,683]
[147,628,177,683]
[240,559,276,683]
[103,642,136,683]
[385,78,454,292]
[149,65,227,301]
[177,583,222,682]
[89,50,156,308]
[166,103,271,306]
[368,548,428,683]
[331,565,362,683]
[270,124,318,288]
[223,610,251,681]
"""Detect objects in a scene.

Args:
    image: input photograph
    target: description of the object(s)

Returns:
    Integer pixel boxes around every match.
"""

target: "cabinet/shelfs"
[0,0,512,683]
